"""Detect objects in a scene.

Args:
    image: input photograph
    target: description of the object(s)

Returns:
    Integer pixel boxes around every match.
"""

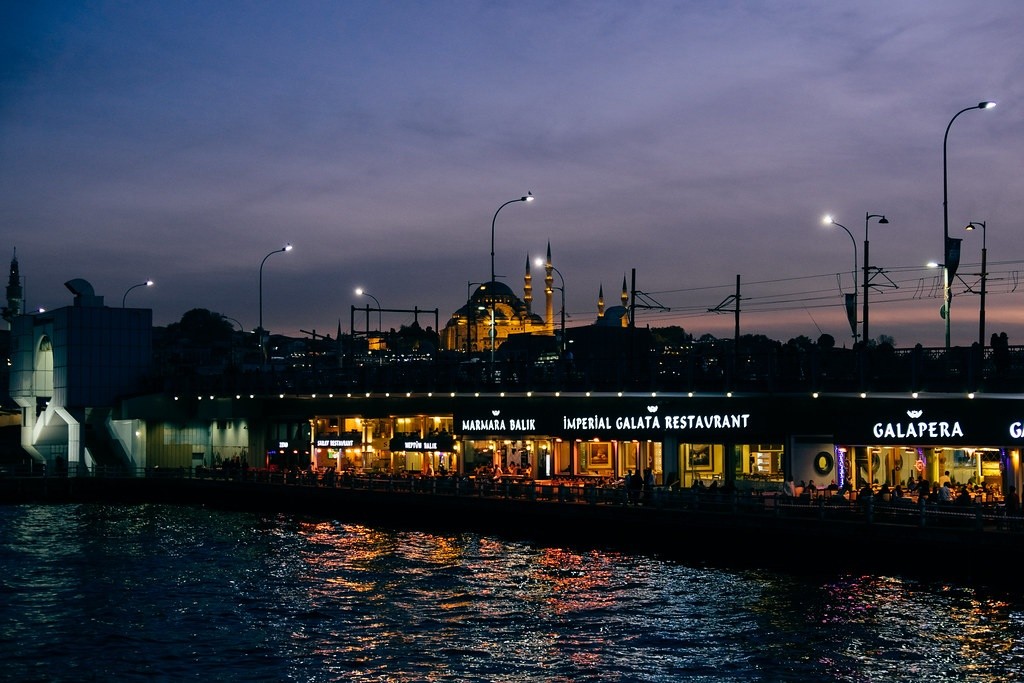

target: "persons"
[201,428,1021,509]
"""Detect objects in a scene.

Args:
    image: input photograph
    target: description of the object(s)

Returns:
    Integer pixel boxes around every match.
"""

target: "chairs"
[785,482,1008,520]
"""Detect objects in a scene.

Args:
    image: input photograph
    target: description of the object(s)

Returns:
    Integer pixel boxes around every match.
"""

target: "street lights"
[864,210,889,343]
[824,216,858,343]
[467,280,486,355]
[490,196,535,363]
[533,258,566,343]
[122,281,154,308]
[965,220,988,349]
[259,243,292,365]
[27,308,45,315]
[941,100,996,386]
[355,288,382,351]
[926,262,944,270]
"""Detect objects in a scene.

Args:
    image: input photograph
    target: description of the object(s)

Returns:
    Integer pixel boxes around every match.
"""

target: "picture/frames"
[586,442,613,470]
[684,444,714,472]
[624,443,637,469]
